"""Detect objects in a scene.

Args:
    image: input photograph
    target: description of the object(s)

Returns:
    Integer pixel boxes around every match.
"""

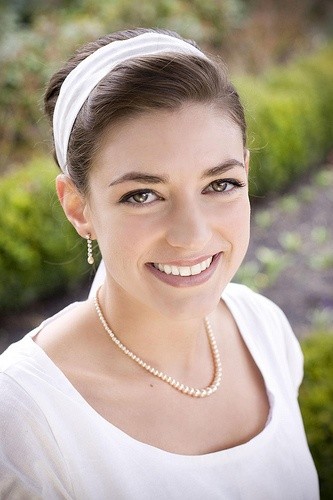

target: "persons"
[1,27,321,499]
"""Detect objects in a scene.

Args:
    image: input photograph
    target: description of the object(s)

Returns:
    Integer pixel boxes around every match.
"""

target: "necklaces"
[95,284,222,398]
